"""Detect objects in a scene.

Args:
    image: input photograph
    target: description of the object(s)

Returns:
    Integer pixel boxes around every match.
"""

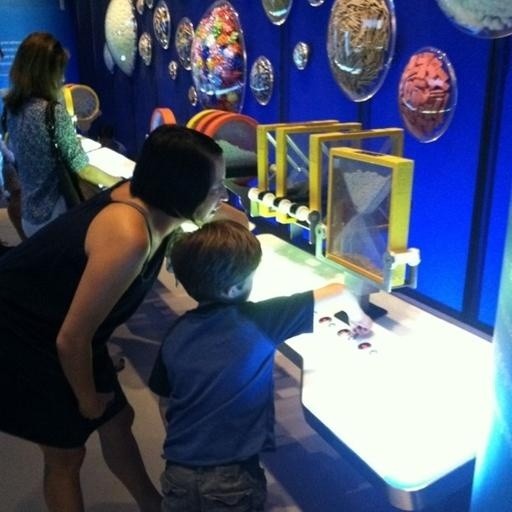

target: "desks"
[70,147,256,249]
[63,133,104,158]
[175,232,499,508]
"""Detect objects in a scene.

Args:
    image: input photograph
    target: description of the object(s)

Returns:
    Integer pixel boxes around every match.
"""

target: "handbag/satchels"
[43,98,103,210]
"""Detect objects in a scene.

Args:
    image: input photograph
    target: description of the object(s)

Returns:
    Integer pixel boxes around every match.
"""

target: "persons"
[0,49,15,207]
[147,219,344,512]
[0,33,125,371]
[0,124,229,512]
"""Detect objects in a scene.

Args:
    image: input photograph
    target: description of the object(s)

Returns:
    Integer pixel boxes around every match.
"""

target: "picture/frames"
[255,124,312,218]
[277,123,363,224]
[309,128,405,229]
[324,147,414,290]
